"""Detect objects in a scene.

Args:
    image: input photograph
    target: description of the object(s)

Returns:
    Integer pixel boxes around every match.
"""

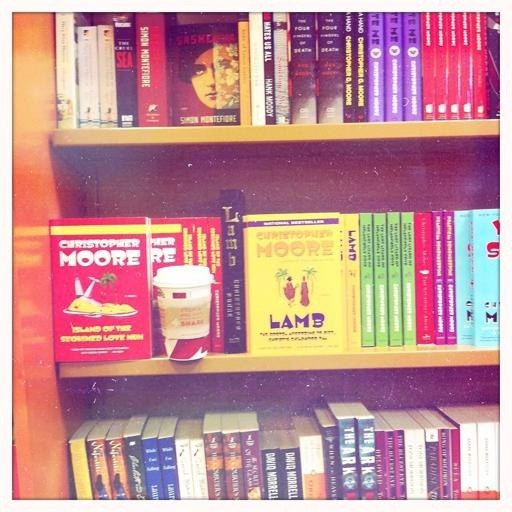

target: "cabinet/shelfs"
[14,115,500,501]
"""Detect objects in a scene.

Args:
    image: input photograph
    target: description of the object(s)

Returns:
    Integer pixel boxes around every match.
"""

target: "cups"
[153,266,214,361]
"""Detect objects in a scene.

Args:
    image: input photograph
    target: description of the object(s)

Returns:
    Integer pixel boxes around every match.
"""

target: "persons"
[178,22,240,124]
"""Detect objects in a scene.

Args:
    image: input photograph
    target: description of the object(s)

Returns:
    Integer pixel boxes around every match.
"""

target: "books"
[48,188,500,363]
[53,11,500,126]
[69,402,501,499]
